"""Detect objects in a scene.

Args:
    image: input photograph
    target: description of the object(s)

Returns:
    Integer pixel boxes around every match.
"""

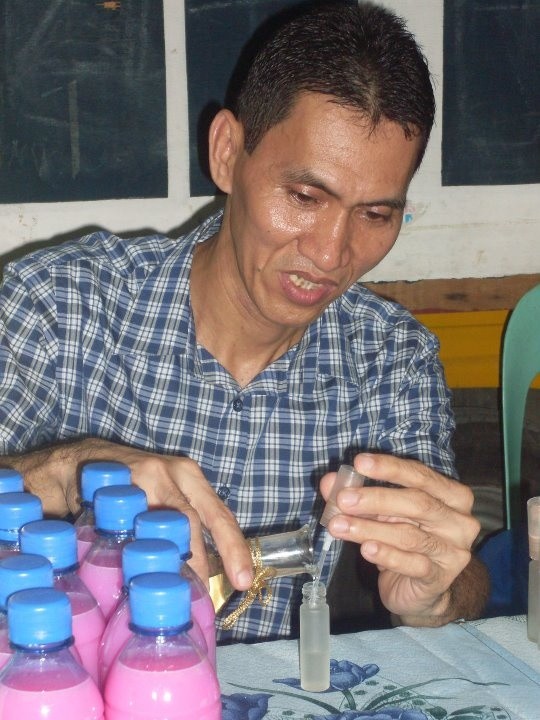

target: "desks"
[216,612,540,720]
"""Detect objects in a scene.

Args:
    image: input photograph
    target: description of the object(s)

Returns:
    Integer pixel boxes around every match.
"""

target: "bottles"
[205,524,316,615]
[299,582,331,691]
[0,459,221,720]
[527,496,540,644]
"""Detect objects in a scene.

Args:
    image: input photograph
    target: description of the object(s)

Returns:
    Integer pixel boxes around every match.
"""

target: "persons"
[1,1,490,645]
